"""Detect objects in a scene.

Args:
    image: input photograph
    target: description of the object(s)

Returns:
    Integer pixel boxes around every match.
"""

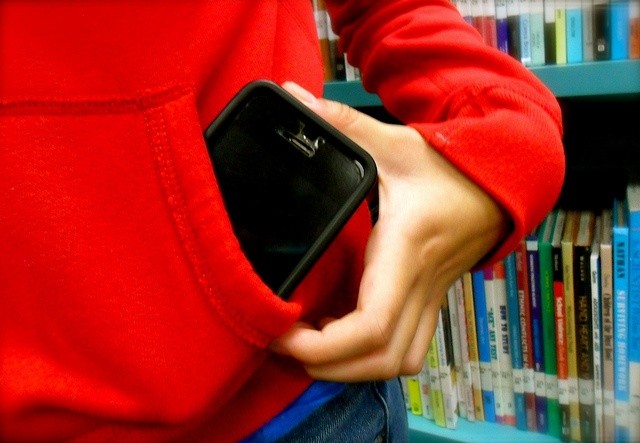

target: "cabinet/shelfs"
[310,0,640,442]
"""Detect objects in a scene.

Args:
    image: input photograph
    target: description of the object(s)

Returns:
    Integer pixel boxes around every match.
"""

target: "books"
[398,183,639,443]
[457,2,639,67]
[313,0,362,82]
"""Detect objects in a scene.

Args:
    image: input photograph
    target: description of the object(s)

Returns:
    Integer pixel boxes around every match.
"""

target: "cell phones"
[201,77,378,303]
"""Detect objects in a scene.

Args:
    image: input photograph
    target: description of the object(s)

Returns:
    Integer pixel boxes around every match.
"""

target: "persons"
[1,1,566,442]
